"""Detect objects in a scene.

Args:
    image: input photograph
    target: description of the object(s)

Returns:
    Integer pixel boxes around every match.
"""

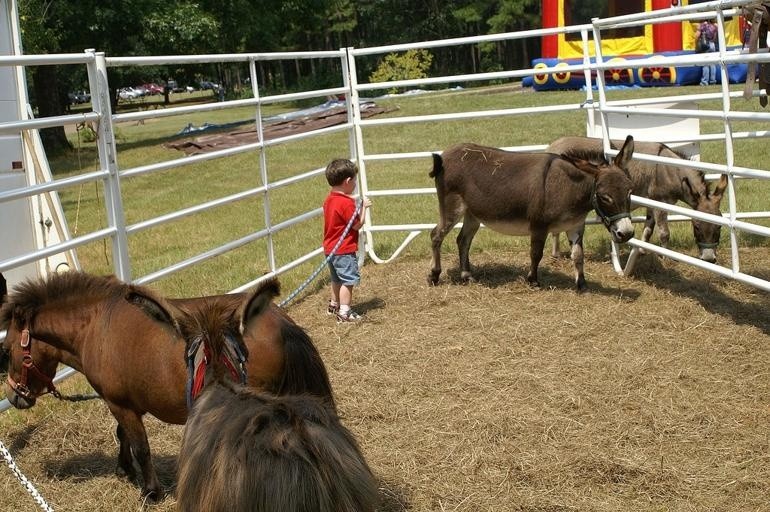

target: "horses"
[1,266,338,504]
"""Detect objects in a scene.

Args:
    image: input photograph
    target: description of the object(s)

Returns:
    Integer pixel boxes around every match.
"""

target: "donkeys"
[425,135,636,297]
[546,135,728,264]
[120,276,382,512]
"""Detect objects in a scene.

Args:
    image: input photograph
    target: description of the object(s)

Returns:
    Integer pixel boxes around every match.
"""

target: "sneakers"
[327,301,340,316]
[337,308,362,322]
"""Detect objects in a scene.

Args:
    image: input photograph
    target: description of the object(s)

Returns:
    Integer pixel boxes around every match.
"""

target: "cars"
[198,82,217,90]
[116,80,194,104]
[67,89,92,107]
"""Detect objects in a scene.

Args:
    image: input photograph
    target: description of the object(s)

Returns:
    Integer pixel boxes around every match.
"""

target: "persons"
[323,159,372,323]
[695,20,718,87]
